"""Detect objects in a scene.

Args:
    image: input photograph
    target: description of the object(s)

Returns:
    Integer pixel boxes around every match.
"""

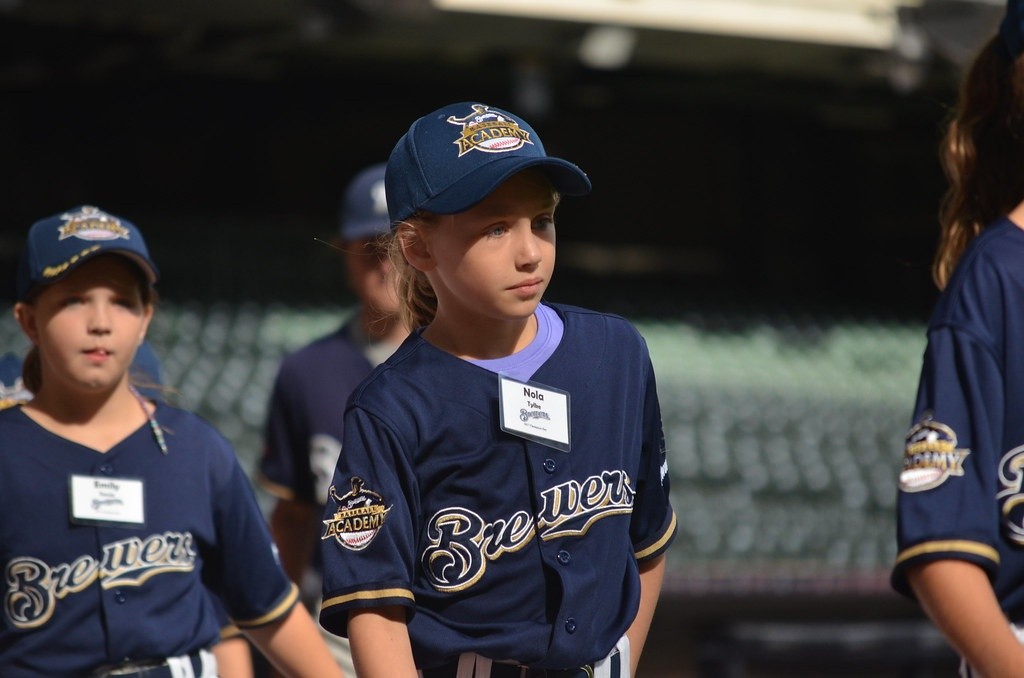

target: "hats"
[341,162,395,238]
[383,101,591,235]
[21,206,158,303]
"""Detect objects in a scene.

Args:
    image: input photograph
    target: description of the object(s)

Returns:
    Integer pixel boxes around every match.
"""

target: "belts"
[424,651,621,678]
[105,650,200,677]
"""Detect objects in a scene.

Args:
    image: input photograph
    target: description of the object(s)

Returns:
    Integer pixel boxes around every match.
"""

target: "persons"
[318,100,678,678]
[0,205,343,678]
[250,164,416,623]
[891,0,1024,678]
